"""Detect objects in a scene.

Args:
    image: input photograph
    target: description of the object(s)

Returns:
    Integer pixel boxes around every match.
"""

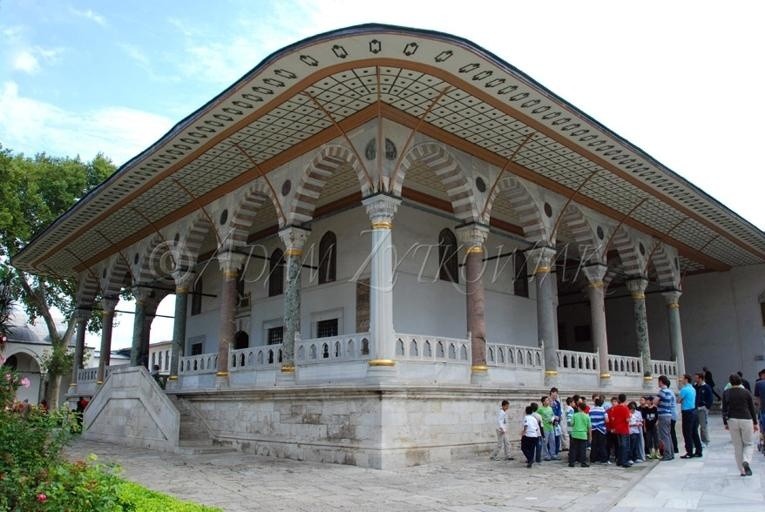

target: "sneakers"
[681,453,702,458]
[741,462,752,476]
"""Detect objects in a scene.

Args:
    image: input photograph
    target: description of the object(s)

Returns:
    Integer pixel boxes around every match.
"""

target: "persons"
[519,373,714,467]
[724,368,764,456]
[488,396,515,463]
[702,366,722,402]
[722,373,760,476]
[136,352,147,366]
[151,363,160,384]
[19,394,92,428]
